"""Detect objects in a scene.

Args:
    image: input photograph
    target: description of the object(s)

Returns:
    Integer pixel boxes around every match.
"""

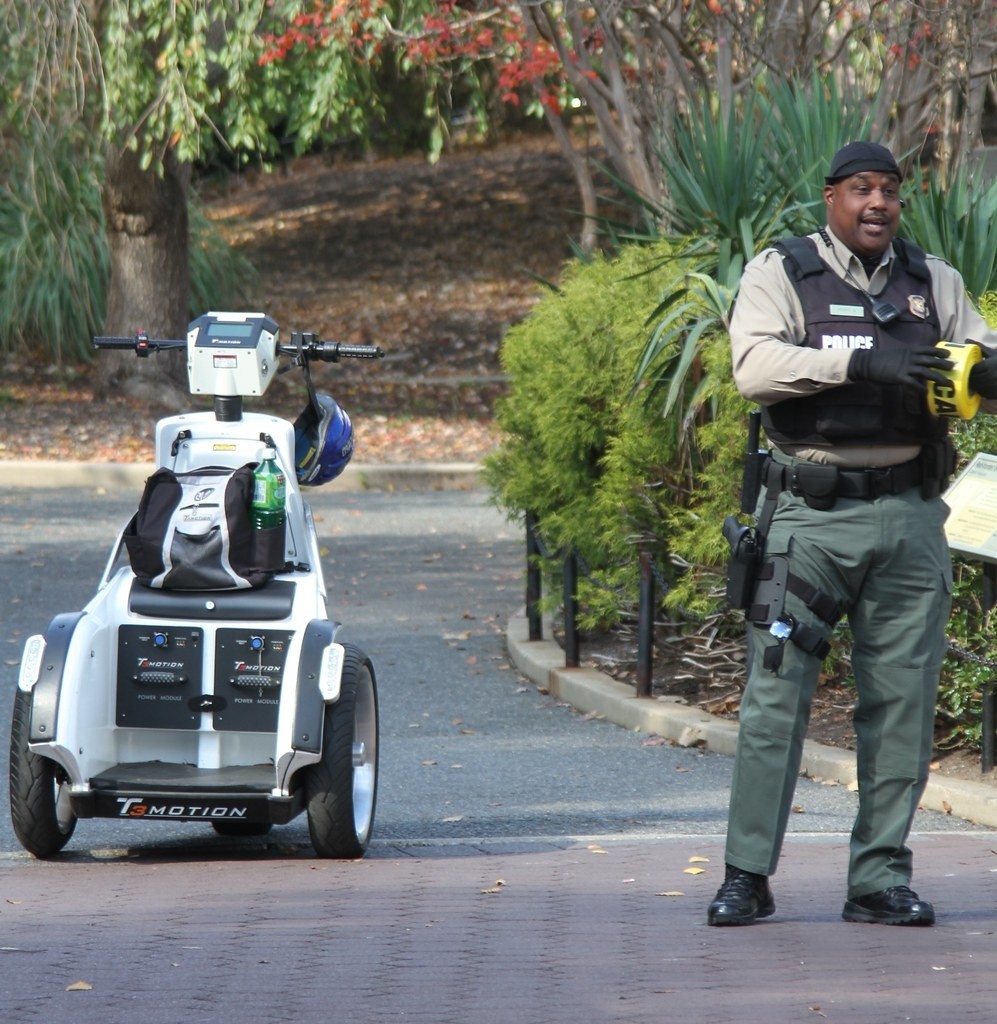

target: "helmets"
[290,391,352,487]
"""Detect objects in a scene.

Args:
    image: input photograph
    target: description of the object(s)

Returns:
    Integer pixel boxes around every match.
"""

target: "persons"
[708,142,997,926]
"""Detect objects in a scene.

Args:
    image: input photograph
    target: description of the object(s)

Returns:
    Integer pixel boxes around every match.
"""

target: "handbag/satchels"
[124,462,288,593]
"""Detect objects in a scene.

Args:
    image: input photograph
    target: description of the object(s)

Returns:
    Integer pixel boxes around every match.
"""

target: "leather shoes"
[705,864,776,923]
[841,886,935,926]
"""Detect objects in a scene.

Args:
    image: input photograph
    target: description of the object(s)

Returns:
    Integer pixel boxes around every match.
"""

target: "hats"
[825,140,904,183]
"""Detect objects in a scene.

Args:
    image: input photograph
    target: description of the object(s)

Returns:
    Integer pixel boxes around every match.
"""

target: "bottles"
[248,447,285,529]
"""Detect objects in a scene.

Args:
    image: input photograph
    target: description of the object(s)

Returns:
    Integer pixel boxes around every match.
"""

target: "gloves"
[853,340,951,392]
[961,337,996,397]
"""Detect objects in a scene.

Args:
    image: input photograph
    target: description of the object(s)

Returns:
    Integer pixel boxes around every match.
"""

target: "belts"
[846,454,922,498]
